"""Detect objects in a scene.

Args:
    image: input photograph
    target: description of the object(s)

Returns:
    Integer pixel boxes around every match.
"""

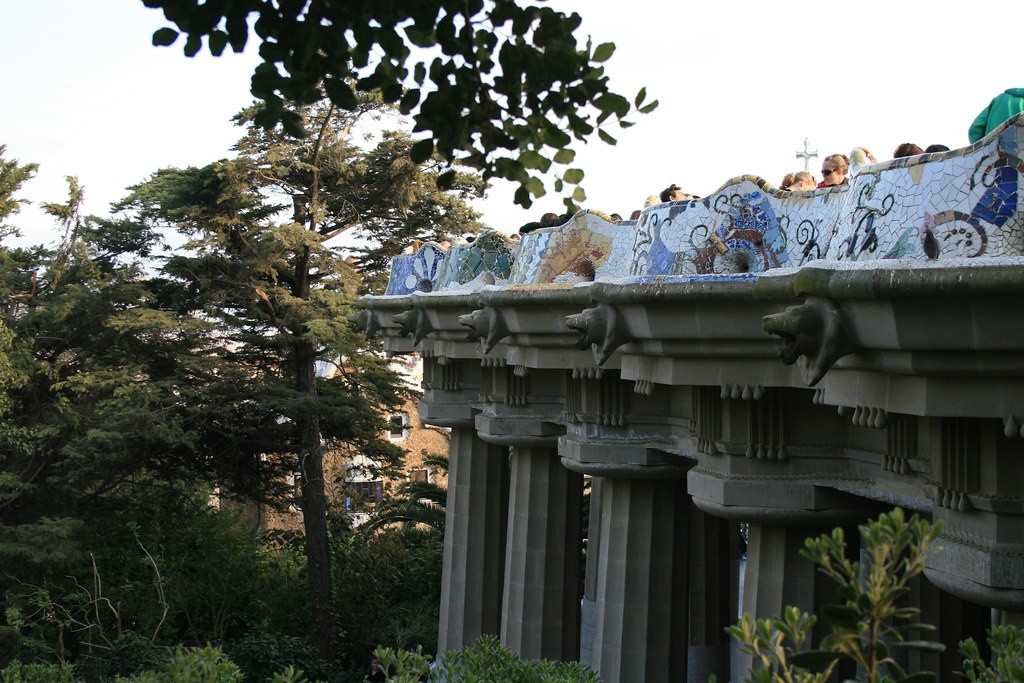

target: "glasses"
[821,169,838,175]
[670,184,674,190]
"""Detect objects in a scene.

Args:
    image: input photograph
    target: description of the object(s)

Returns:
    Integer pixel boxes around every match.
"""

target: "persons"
[364,657,386,683]
[509,88,1024,240]
